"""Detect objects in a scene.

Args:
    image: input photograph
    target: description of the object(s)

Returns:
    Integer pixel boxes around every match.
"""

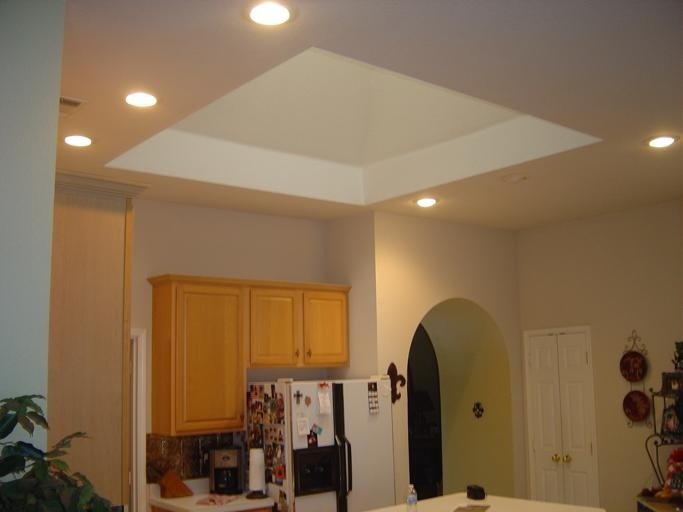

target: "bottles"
[407,484,417,512]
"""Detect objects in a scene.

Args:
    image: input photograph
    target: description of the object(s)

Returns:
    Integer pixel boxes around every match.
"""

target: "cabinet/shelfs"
[247,280,351,367]
[145,273,245,436]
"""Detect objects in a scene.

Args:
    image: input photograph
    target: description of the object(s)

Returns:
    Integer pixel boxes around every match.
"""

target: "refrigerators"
[247,376,397,512]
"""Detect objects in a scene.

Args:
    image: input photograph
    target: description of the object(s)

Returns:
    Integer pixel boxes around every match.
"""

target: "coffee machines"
[209,448,241,494]
